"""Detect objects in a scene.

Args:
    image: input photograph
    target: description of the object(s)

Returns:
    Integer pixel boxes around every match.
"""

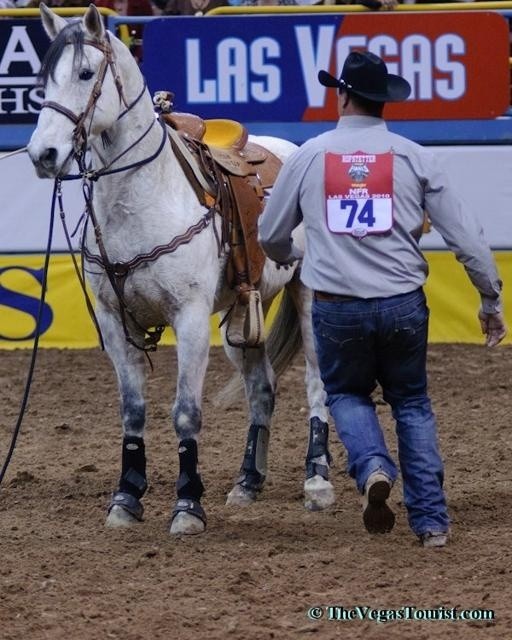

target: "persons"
[258,49,507,549]
[2,1,399,17]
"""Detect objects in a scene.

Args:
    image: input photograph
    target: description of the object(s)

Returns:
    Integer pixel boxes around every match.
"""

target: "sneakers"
[418,531,447,547]
[363,473,395,533]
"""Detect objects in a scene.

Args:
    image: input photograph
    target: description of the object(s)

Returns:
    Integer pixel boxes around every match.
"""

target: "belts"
[312,289,359,304]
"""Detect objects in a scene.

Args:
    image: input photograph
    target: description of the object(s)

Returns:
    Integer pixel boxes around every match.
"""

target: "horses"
[25,1,336,536]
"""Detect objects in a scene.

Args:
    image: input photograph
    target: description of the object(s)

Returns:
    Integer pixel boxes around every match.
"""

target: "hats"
[319,52,410,102]
